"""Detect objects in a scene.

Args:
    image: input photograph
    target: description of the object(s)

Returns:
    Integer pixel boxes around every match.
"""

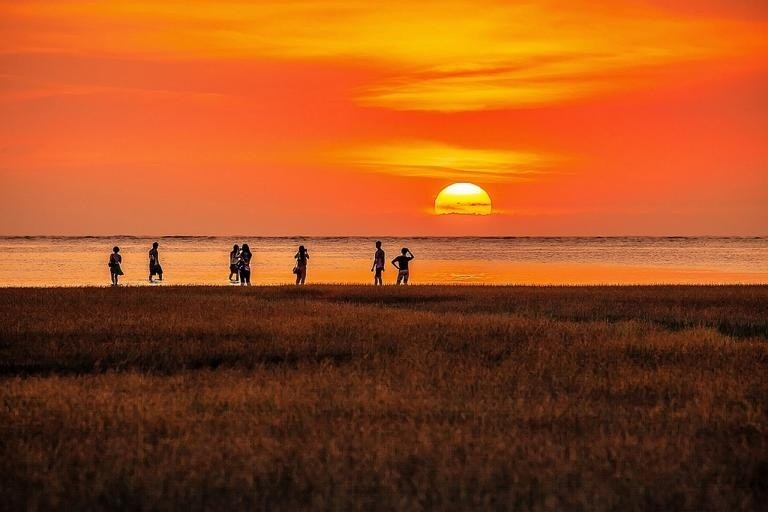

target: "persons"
[109,246,124,286]
[149,241,164,280]
[391,247,414,285]
[235,244,252,285]
[229,244,239,281]
[294,245,309,285]
[371,241,384,286]
